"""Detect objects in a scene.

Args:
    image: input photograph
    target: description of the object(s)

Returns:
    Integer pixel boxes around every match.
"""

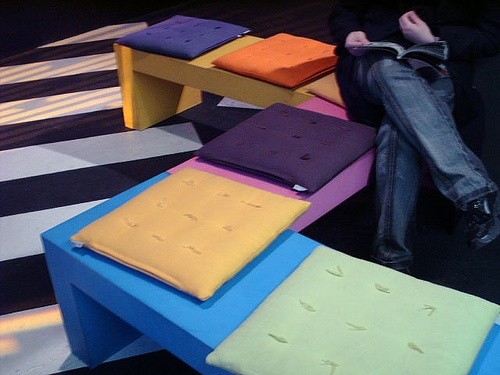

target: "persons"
[329,0,500,276]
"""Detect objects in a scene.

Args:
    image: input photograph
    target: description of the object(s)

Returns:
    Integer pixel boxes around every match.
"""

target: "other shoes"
[465,188,500,248]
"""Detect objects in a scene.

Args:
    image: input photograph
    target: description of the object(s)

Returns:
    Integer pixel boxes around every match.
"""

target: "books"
[351,38,453,78]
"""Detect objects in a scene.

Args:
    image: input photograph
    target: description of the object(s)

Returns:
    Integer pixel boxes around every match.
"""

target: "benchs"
[40,14,500,375]
